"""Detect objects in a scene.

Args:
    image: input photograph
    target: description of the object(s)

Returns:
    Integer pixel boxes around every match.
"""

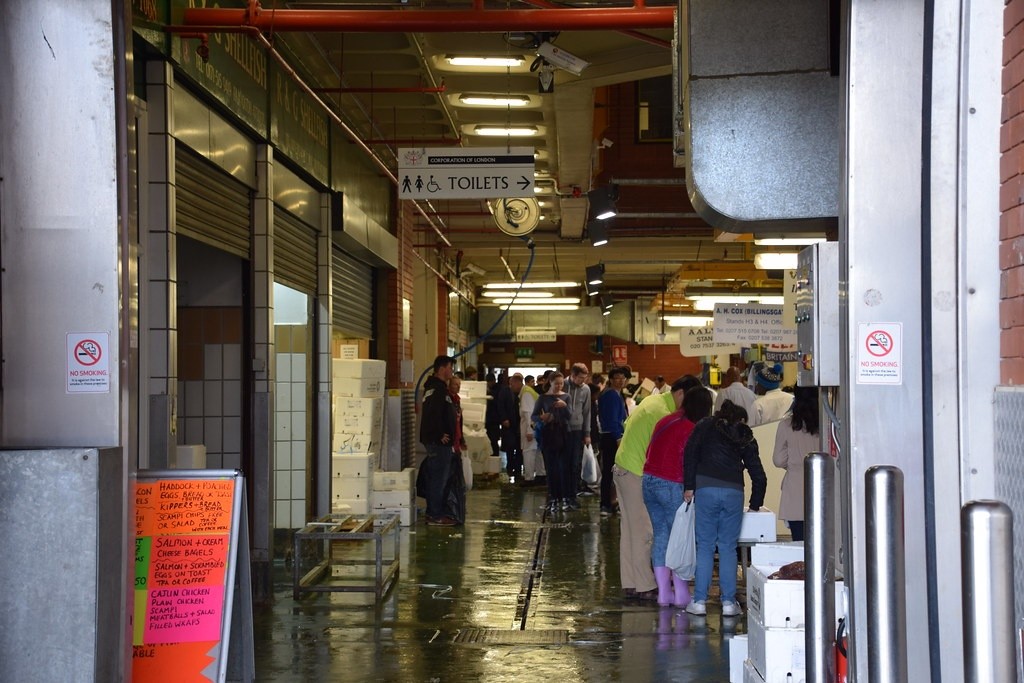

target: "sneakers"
[686,598,707,615]
[722,600,744,615]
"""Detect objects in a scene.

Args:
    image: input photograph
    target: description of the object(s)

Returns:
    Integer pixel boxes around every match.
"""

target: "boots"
[652,566,691,608]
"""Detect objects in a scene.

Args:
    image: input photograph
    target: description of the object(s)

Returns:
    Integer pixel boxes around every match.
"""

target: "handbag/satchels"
[664,495,696,578]
[580,443,598,483]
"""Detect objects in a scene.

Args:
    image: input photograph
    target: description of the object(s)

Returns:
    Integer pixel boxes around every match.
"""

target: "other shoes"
[424,514,459,525]
[633,588,658,600]
[599,506,613,515]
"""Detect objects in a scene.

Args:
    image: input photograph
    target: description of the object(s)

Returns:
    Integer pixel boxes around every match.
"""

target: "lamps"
[585,264,605,285]
[587,187,616,220]
[600,306,610,316]
[586,219,607,247]
[585,282,599,296]
[600,294,613,308]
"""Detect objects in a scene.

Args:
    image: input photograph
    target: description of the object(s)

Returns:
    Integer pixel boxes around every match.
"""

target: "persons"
[418,355,820,617]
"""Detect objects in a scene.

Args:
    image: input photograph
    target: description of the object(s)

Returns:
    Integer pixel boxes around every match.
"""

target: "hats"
[756,363,783,390]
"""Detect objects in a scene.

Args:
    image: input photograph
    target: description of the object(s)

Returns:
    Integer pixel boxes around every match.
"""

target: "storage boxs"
[178,445,206,469]
[729,504,845,683]
[330,356,502,527]
